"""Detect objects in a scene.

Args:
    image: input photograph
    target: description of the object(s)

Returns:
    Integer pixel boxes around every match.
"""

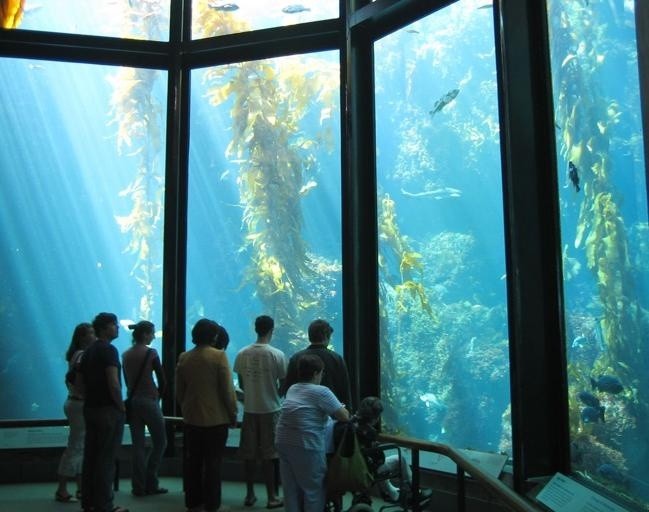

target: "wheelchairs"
[326,391,433,512]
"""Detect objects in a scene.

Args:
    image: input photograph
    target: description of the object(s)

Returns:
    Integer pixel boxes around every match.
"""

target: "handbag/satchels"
[123,399,131,424]
[326,452,376,494]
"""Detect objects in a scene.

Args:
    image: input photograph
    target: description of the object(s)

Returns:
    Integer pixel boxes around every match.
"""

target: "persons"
[285,319,353,419]
[122,321,168,496]
[274,354,349,512]
[55,322,97,503]
[174,319,238,512]
[348,397,433,503]
[234,316,287,509]
[79,313,129,511]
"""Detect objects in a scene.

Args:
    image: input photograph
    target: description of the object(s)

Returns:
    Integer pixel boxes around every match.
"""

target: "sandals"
[112,505,130,511]
[84,505,101,511]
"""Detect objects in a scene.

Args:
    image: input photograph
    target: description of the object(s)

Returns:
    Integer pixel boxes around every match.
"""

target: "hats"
[308,319,334,338]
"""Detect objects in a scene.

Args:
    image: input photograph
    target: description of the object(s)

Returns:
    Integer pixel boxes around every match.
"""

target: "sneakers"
[150,486,169,494]
[54,491,78,503]
[383,486,401,502]
[411,486,433,509]
[131,488,149,497]
[265,499,282,509]
[244,494,256,506]
